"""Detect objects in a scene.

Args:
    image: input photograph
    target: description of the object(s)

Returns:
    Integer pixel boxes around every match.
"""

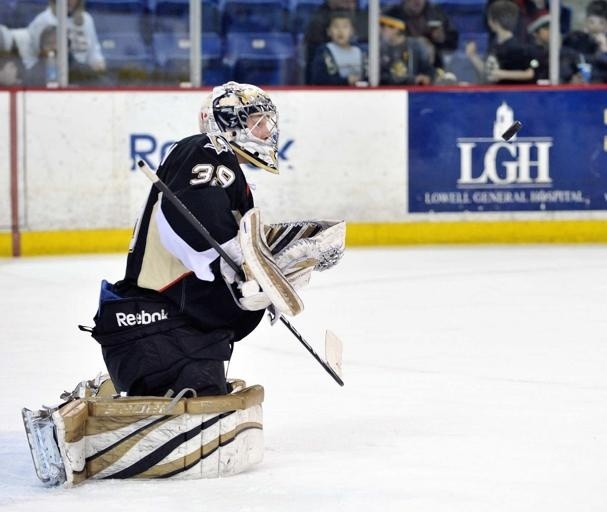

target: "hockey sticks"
[137,160,345,388]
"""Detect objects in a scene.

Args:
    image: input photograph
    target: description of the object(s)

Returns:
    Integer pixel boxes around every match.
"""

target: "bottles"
[43,51,59,89]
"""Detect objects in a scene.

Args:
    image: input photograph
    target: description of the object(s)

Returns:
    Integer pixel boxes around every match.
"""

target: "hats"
[378,6,409,31]
[524,7,549,37]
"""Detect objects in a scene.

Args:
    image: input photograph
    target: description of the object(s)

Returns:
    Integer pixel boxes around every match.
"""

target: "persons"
[19,79,347,485]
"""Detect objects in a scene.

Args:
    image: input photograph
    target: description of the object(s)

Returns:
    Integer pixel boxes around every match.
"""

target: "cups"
[576,63,592,85]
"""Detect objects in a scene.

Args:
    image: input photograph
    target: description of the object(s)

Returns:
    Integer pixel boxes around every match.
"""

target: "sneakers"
[32,404,66,486]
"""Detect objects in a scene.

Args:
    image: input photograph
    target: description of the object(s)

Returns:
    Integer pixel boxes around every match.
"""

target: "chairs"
[447,3,488,56]
[202,3,321,85]
[87,2,189,84]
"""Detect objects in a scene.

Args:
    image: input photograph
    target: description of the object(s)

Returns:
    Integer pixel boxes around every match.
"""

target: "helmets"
[198,80,280,153]
